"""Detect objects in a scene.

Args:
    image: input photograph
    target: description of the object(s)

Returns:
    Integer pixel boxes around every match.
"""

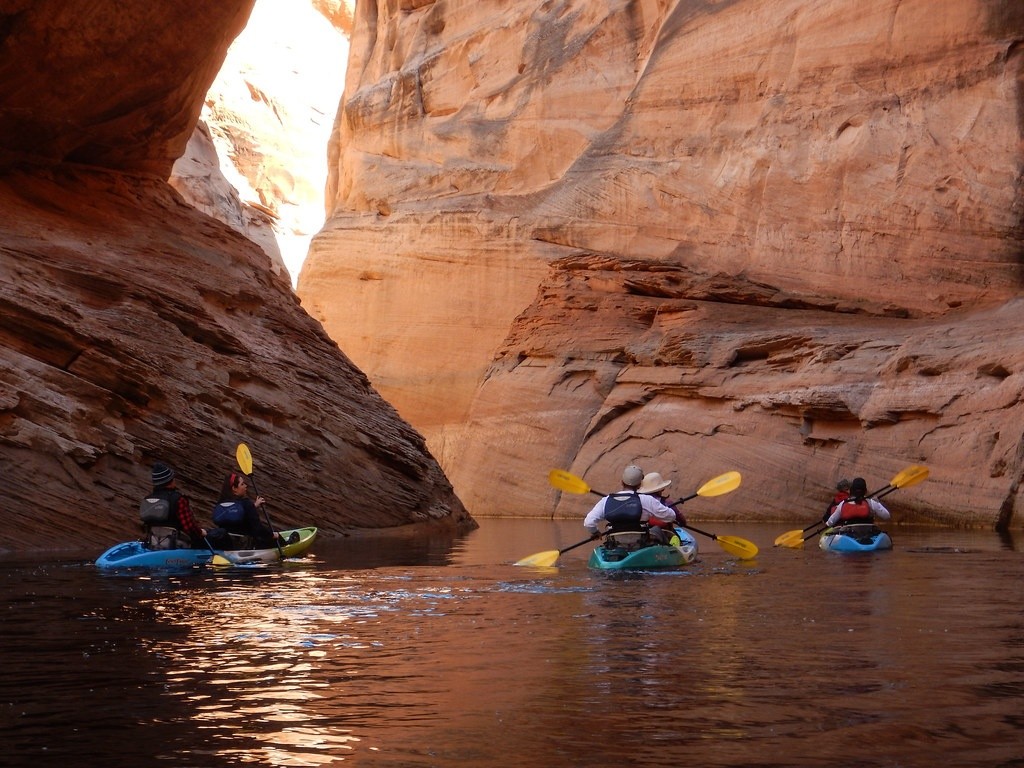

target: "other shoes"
[289,531,300,543]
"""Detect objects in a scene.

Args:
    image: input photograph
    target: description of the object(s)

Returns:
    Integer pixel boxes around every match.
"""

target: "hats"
[849,477,868,496]
[622,466,644,486]
[635,472,672,494]
[151,462,174,485]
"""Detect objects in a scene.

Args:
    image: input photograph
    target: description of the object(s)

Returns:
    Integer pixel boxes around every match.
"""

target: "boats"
[588,527,698,571]
[93,527,320,574]
[820,529,894,552]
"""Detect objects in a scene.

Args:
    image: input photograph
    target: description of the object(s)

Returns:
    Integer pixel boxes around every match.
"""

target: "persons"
[823,477,890,534]
[217,471,300,550]
[584,466,687,546]
[147,463,255,551]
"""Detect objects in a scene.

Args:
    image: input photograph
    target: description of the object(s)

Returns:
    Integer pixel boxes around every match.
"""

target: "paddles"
[518,470,742,568]
[784,467,930,550]
[204,537,231,565]
[548,468,759,560]
[776,465,920,547]
[236,443,284,560]
